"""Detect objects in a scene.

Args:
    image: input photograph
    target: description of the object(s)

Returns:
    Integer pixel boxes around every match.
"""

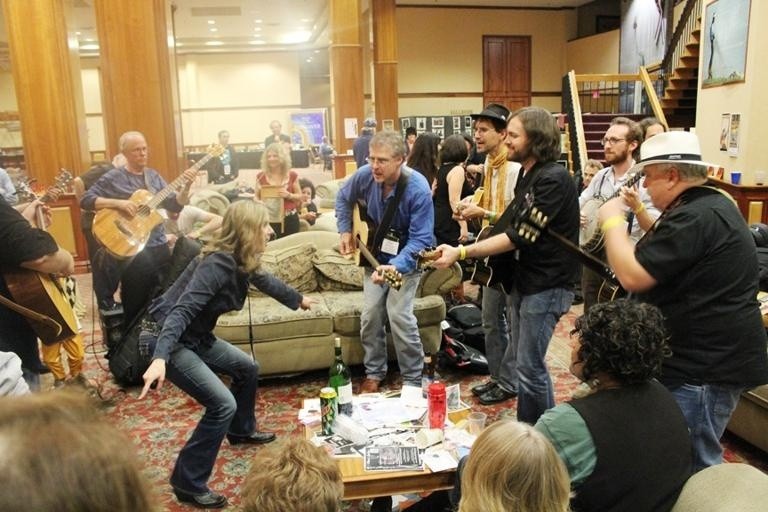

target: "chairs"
[190,189,231,239]
[297,173,352,231]
[724,383,768,455]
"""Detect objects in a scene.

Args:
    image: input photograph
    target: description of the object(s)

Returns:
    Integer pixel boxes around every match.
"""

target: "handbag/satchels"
[439,300,488,374]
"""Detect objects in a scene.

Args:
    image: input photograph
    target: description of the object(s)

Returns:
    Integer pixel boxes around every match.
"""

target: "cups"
[428,383,446,430]
[468,411,487,436]
[731,172,741,184]
[320,386,337,436]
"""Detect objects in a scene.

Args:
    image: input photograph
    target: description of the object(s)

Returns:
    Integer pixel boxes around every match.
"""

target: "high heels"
[226,430,275,444]
[173,489,226,508]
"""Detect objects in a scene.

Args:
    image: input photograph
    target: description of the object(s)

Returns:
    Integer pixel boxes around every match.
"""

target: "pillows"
[246,241,365,297]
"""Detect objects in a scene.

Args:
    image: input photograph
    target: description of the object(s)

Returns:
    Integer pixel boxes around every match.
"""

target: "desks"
[187,147,310,171]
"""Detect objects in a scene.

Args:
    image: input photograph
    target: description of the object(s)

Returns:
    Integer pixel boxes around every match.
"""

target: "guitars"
[91,145,228,260]
[351,196,402,291]
[418,224,509,292]
[4,169,78,343]
[501,196,628,302]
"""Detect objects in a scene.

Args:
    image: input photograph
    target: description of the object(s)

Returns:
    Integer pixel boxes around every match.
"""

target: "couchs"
[212,230,464,380]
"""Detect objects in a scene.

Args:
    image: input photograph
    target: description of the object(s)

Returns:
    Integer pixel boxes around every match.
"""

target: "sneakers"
[72,373,95,393]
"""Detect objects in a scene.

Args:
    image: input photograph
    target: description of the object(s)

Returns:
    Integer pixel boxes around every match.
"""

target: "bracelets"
[602,216,623,230]
[632,202,645,215]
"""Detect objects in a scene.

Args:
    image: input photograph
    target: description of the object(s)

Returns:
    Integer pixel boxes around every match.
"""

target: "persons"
[577,116,664,314]
[534,298,697,512]
[137,198,318,507]
[248,120,337,238]
[239,439,344,511]
[0,390,168,512]
[1,167,94,391]
[334,102,581,423]
[601,130,768,474]
[401,422,572,512]
[73,131,240,325]
[635,116,667,169]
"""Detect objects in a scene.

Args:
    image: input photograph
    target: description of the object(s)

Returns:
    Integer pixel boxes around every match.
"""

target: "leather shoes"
[471,381,518,405]
[361,378,383,395]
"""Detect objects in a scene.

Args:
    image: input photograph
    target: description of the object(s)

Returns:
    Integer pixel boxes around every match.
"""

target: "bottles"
[422,350,435,398]
[327,337,353,417]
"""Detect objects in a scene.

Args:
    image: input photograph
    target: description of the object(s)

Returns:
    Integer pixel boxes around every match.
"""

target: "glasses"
[366,153,403,168]
[601,137,628,147]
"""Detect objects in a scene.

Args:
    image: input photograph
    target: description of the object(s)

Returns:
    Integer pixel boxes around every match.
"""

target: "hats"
[470,104,510,124]
[626,130,720,176]
